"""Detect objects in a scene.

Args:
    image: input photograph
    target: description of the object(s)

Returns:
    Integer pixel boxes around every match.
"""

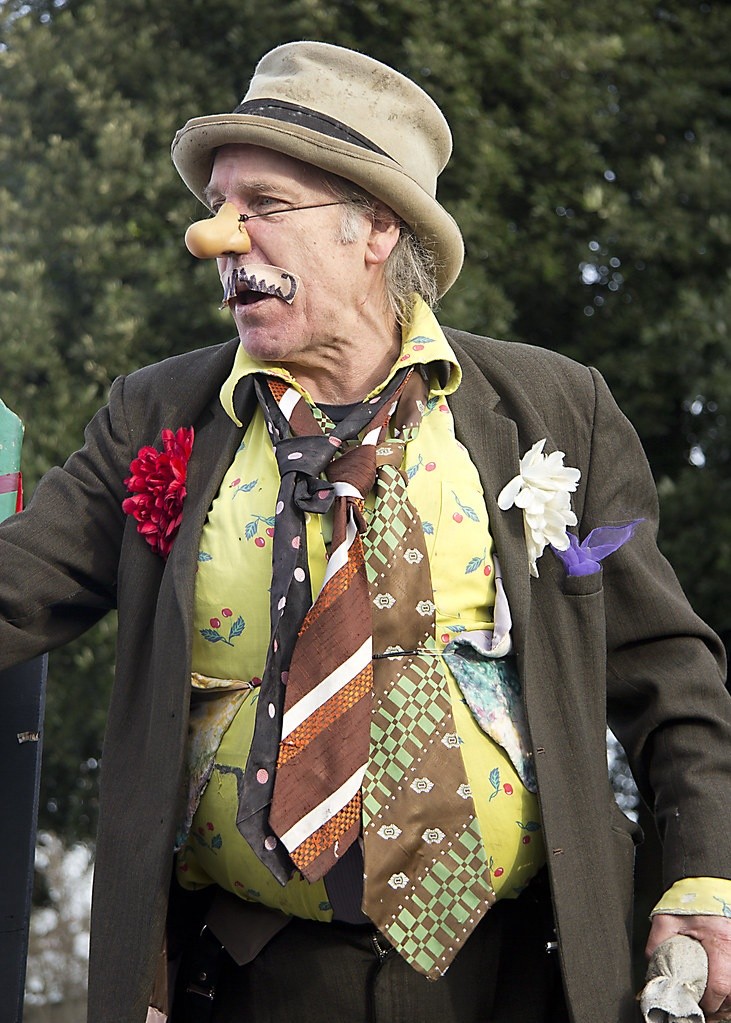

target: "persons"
[0,41,731,1023]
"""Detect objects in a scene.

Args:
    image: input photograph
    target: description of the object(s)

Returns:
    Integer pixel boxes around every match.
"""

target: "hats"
[170,41,464,300]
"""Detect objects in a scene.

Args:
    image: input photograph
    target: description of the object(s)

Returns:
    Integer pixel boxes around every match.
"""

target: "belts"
[288,866,553,960]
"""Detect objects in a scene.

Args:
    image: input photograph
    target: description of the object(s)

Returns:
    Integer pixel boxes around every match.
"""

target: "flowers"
[499,437,583,578]
[121,424,195,559]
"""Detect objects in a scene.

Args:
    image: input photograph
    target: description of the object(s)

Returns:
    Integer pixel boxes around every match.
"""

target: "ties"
[236,361,415,887]
[362,362,497,984]
